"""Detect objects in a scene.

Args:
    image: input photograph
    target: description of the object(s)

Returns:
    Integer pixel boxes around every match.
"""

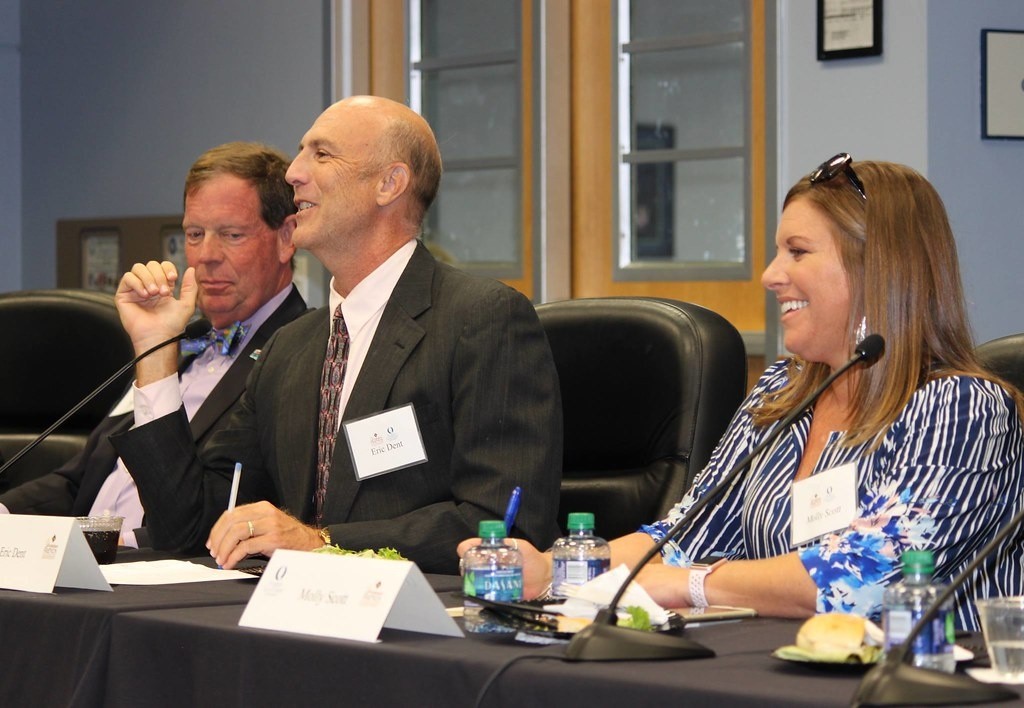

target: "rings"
[512,539,517,550]
[248,520,254,537]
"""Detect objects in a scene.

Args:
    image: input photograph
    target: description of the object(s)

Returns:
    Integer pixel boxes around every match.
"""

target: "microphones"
[0,316,212,476]
[567,332,887,659]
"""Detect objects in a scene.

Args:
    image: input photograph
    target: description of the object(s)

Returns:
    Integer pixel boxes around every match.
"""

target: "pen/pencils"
[217,463,242,569]
[504,485,522,538]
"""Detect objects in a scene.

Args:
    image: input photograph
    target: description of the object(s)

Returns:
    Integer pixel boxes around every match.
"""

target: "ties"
[306,303,349,528]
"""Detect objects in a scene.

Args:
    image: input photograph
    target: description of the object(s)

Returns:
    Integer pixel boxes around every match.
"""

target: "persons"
[107,95,562,575]
[457,151,1024,631]
[0,142,316,549]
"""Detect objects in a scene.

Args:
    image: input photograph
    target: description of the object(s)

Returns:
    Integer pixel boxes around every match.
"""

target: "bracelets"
[319,527,331,545]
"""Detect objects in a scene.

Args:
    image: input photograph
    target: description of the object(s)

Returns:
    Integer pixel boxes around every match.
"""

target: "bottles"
[552,512,611,593]
[883,552,955,675]
[464,521,523,633]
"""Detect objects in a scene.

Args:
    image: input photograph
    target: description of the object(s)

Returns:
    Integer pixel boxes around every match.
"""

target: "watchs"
[689,556,728,608]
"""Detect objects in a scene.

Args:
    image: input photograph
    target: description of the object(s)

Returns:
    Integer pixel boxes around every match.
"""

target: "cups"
[975,598,1024,679]
[74,517,125,565]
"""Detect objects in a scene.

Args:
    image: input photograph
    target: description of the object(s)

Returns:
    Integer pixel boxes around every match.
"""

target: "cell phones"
[670,605,758,623]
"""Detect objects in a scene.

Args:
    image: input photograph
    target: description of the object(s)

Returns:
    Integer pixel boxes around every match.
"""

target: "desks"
[0,549,1024,707]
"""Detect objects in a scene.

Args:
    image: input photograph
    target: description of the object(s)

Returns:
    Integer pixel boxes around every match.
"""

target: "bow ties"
[178,321,241,357]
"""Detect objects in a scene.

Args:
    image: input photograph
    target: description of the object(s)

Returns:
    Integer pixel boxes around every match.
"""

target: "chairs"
[529,296,747,556]
[0,290,138,501]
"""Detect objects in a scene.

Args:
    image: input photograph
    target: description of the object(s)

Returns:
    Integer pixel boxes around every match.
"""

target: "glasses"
[811,152,864,200]
